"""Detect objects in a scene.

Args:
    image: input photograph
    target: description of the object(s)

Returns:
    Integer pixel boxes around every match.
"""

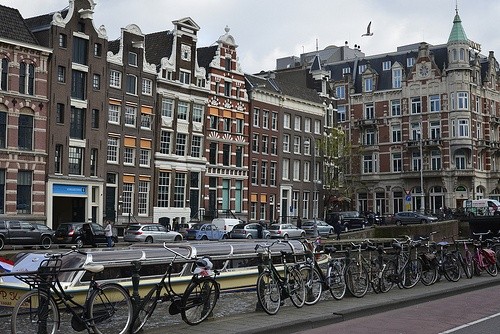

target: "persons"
[334,221,341,240]
[104,220,112,247]
[296,216,302,229]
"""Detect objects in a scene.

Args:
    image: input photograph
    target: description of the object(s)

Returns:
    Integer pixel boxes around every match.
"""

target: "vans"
[212,218,243,235]
[463,199,500,218]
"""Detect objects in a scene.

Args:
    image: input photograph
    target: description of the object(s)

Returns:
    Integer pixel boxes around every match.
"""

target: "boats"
[0,251,332,308]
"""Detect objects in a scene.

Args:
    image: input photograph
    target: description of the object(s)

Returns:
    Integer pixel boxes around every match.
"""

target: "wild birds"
[361,21,373,36]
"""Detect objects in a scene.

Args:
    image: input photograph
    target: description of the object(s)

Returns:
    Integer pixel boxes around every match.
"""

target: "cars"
[302,220,334,237]
[56,223,118,249]
[230,222,270,239]
[0,220,56,251]
[123,224,183,244]
[185,224,224,241]
[269,224,306,239]
[393,211,438,226]
[325,211,367,231]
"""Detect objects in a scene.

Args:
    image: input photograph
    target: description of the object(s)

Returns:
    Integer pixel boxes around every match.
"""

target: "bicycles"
[127,241,221,332]
[253,230,500,315]
[11,249,132,334]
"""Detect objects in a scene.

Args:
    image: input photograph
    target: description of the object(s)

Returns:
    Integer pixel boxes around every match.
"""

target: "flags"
[0,256,15,273]
